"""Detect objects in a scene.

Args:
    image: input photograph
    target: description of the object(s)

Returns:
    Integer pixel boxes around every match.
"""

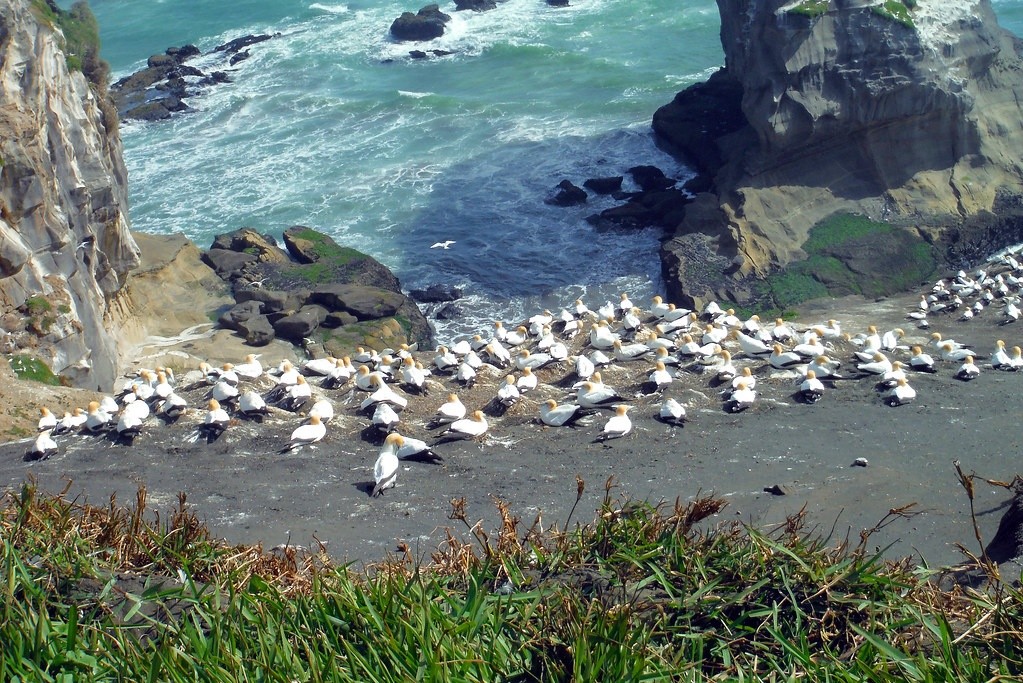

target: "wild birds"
[31,247,1023,499]
[430,240,456,250]
